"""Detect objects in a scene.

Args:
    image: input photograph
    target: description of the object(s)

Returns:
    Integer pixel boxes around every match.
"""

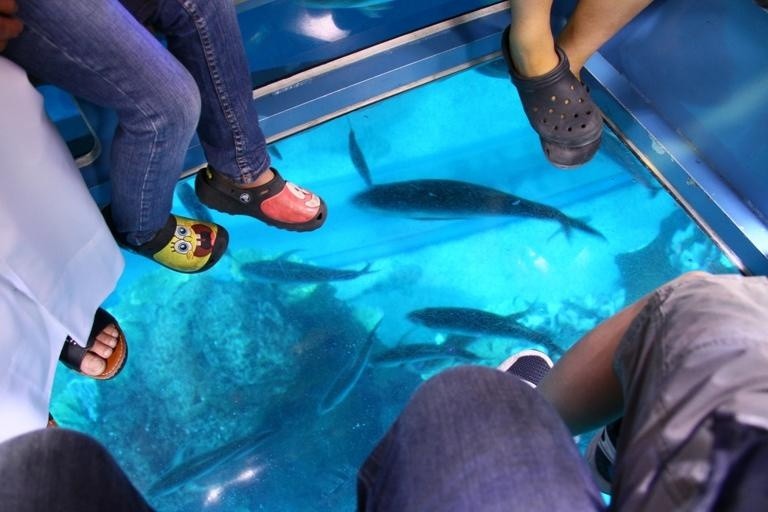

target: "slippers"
[195,163,327,233]
[499,22,604,148]
[103,202,229,273]
[541,137,602,165]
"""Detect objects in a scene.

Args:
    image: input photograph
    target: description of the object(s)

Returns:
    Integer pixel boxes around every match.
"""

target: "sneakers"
[585,425,620,497]
[497,348,554,388]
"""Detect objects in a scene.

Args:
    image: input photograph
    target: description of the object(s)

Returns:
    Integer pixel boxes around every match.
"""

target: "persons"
[1,0,327,275]
[501,0,654,169]
[0,57,129,442]
[0,366,607,512]
[495,270,767,511]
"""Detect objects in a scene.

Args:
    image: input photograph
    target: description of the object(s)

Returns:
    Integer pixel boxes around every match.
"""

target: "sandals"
[59,307,128,381]
